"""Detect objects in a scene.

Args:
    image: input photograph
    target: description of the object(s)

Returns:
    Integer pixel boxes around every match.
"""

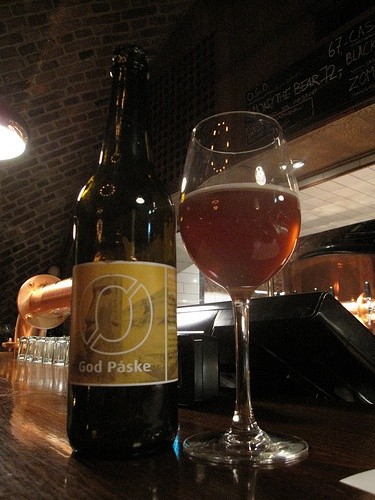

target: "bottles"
[65,51,178,461]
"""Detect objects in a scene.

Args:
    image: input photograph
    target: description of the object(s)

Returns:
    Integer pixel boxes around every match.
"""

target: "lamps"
[0,104,30,160]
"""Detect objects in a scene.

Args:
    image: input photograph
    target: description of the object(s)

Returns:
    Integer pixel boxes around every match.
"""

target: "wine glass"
[177,110,310,470]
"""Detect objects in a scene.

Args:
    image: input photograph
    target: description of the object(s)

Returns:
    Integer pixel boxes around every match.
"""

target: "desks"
[0,350,375,500]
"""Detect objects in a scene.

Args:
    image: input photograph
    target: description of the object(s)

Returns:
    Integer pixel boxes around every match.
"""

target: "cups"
[18,335,70,368]
